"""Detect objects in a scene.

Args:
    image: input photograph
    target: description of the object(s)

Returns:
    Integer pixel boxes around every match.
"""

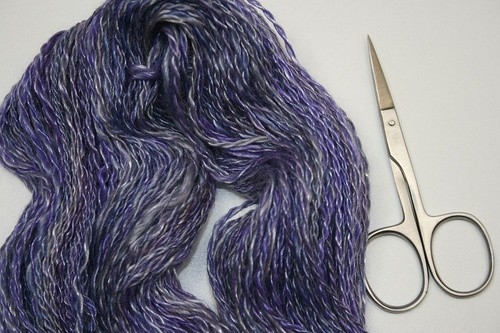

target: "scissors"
[365,33,494,314]
